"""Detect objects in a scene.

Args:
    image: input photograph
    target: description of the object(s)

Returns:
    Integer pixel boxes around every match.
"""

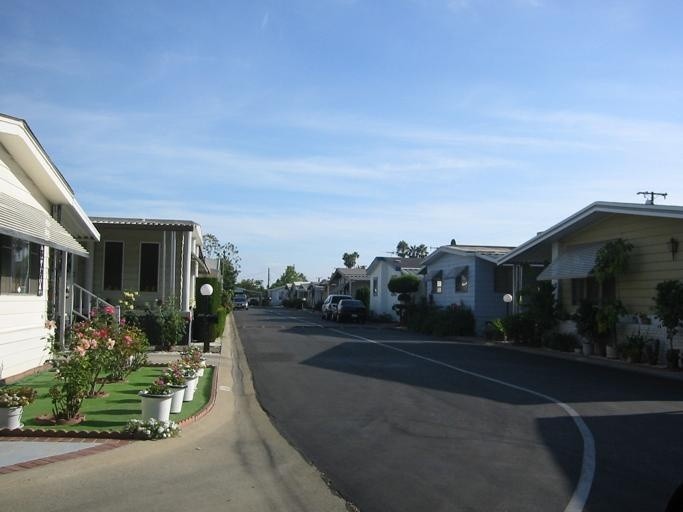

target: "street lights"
[200,283,214,354]
[301,297,307,312]
[502,294,513,340]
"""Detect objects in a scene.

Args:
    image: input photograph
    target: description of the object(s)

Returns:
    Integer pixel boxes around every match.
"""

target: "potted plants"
[144,297,187,351]
[573,280,682,371]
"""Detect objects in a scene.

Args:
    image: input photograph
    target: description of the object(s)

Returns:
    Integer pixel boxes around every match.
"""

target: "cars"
[332,299,368,324]
[233,295,247,310]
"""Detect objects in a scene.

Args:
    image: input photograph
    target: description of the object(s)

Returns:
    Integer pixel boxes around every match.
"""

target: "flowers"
[137,344,206,395]
[124,416,180,441]
[0,386,38,407]
[117,289,139,316]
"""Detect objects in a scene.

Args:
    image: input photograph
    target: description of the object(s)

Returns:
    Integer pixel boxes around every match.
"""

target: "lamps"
[666,237,678,263]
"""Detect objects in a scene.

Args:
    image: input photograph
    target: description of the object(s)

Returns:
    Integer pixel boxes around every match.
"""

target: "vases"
[137,377,195,422]
[119,316,125,323]
[0,406,23,431]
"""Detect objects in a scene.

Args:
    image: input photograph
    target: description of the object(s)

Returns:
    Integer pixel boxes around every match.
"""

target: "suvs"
[320,295,352,321]
